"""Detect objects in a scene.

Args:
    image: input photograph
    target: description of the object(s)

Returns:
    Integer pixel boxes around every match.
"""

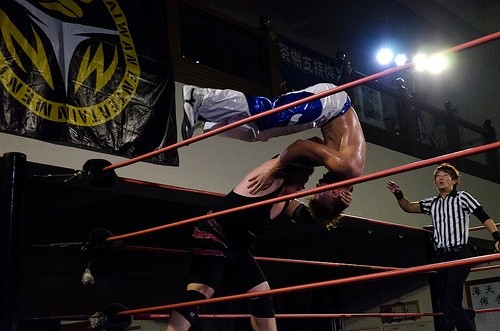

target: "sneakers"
[180,84,202,141]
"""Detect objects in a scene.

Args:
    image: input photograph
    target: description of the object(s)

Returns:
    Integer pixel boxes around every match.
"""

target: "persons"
[387,163,500,331]
[181,83,367,225]
[166,155,353,331]
